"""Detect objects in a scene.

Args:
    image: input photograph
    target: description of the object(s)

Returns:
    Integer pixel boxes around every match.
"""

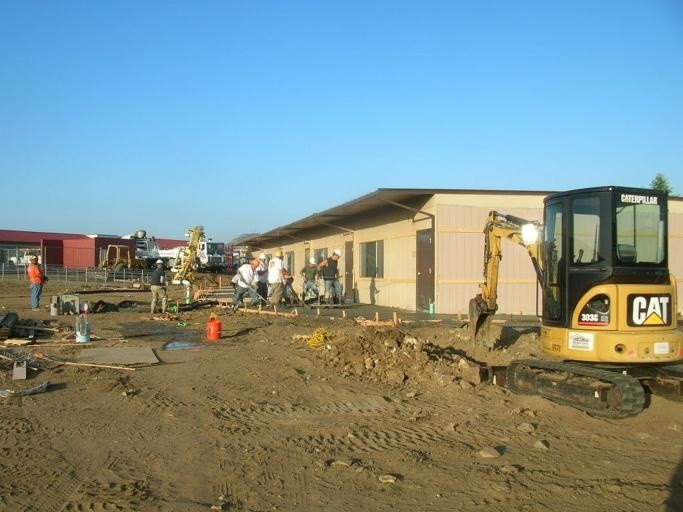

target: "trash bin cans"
[77,315,90,342]
[49,294,59,316]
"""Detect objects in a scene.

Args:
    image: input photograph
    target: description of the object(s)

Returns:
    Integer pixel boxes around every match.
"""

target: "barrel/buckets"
[206,320,222,340]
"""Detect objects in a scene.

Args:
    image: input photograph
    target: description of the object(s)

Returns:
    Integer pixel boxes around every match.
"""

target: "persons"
[151,260,169,314]
[27,255,49,311]
[232,248,342,313]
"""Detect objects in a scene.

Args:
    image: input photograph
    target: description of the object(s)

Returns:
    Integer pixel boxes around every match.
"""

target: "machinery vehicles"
[102,244,132,271]
[468,185,683,420]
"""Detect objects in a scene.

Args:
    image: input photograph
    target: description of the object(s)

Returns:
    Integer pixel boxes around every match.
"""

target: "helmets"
[29,255,37,260]
[155,259,163,264]
[309,257,316,265]
[334,249,342,257]
[250,251,283,267]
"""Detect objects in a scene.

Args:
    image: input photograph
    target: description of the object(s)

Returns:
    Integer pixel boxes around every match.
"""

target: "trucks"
[134,226,227,273]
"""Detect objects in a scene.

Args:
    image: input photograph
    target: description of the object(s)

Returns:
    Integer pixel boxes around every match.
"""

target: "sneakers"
[31,306,41,312]
